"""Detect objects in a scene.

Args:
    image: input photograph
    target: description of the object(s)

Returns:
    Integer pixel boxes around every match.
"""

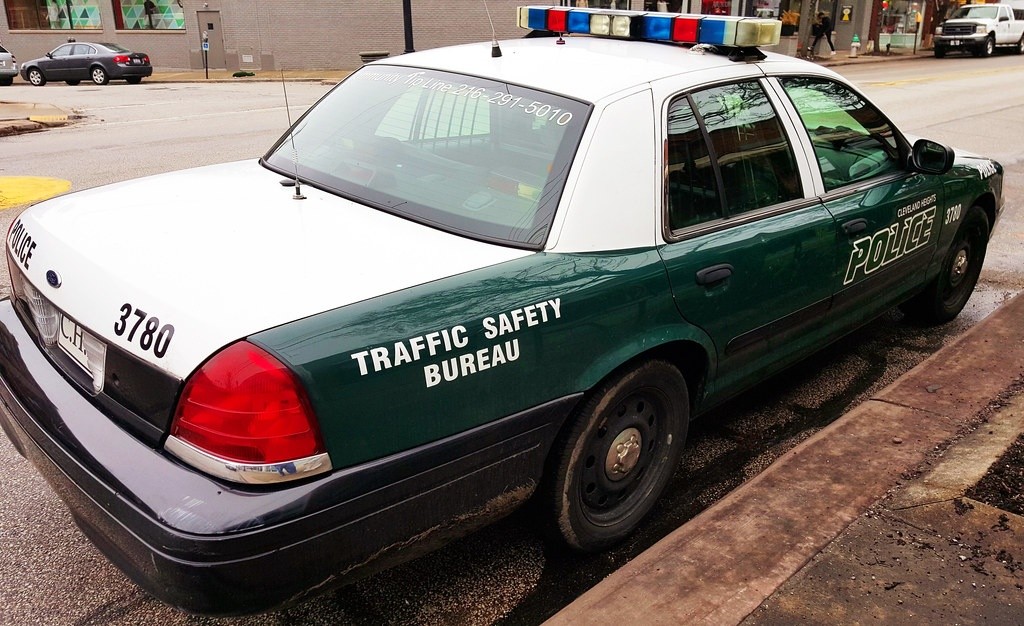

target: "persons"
[806,11,836,56]
[915,11,921,29]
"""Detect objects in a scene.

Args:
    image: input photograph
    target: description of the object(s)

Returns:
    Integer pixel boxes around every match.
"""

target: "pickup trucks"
[933,3,1024,59]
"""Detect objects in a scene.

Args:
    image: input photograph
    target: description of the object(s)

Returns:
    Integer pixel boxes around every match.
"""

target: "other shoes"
[807,46,814,52]
[830,51,836,56]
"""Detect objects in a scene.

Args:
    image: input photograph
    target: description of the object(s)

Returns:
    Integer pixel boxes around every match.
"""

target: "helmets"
[67,37,75,43]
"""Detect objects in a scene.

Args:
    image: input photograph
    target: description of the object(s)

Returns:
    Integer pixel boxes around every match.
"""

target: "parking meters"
[912,10,921,54]
[202,30,210,79]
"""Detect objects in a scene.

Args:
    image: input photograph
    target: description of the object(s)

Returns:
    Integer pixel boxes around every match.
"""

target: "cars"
[0,45,18,86]
[0,7,1007,620]
[19,40,153,84]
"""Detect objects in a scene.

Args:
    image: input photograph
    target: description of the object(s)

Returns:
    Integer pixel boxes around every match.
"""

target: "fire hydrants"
[847,34,862,59]
